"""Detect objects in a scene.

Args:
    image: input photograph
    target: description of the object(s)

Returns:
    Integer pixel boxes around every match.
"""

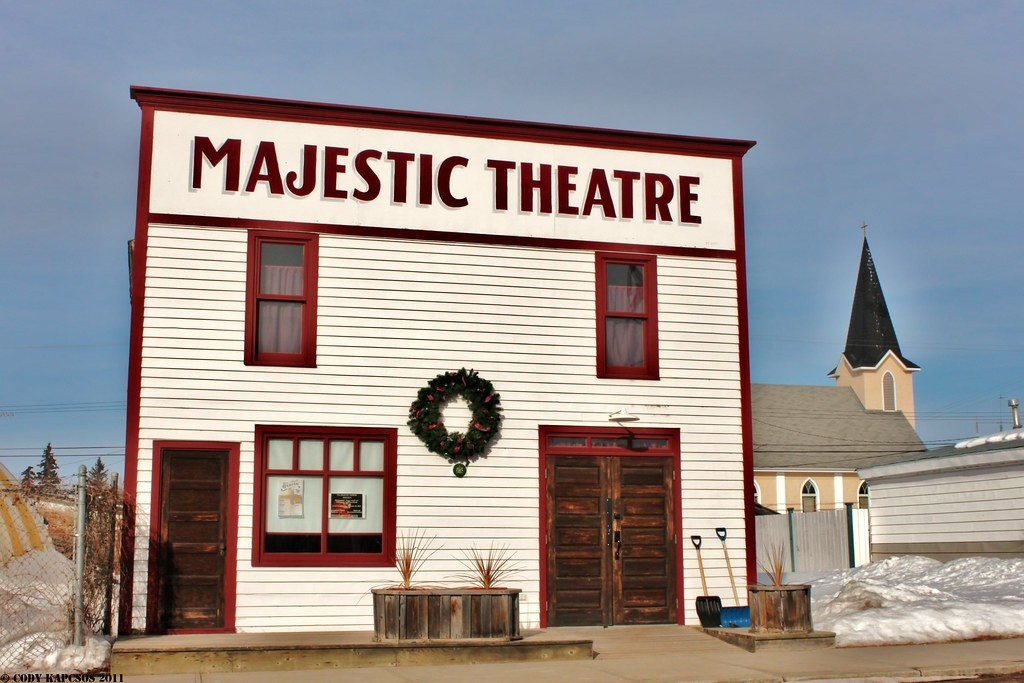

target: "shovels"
[715,527,751,627]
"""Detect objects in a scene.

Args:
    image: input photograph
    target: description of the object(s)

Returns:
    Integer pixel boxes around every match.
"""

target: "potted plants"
[746,540,814,635]
[371,526,524,642]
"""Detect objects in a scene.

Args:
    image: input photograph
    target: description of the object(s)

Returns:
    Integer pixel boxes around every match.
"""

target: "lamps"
[608,407,640,422]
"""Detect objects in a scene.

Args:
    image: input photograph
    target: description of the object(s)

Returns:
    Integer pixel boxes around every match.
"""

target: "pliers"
[690,535,722,628]
